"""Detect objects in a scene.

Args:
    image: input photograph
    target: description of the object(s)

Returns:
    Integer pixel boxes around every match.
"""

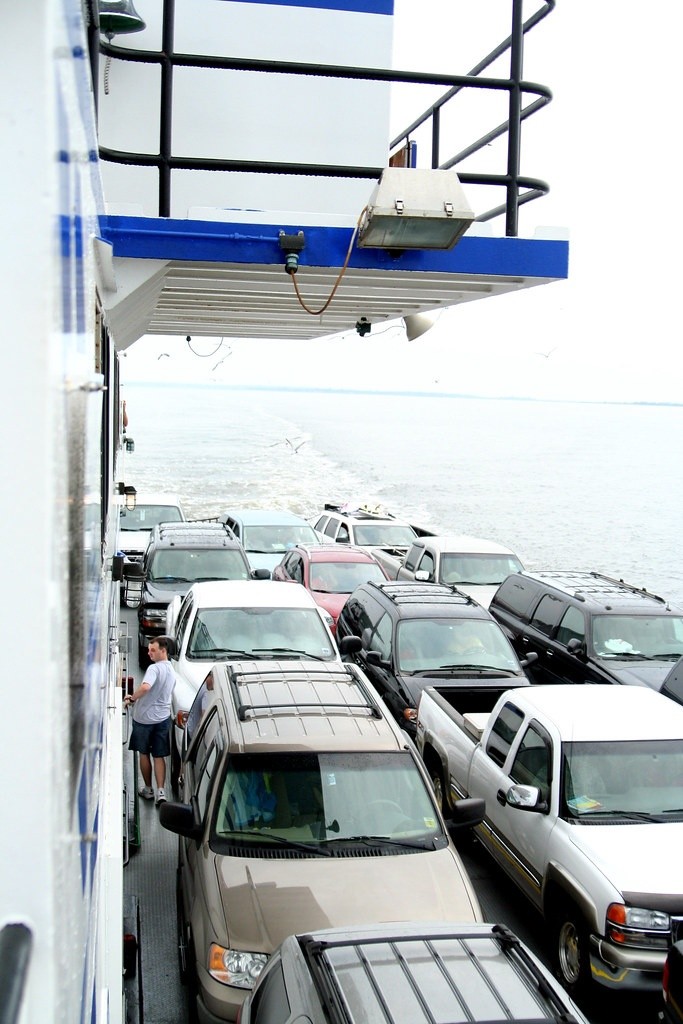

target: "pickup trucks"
[415,681,683,998]
[370,533,528,611]
[165,577,363,794]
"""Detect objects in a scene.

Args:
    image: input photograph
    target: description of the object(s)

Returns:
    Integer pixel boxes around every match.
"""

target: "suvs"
[230,921,593,1024]
[160,660,486,1024]
[116,492,186,570]
[274,544,393,642]
[136,519,272,673]
[218,511,323,580]
[302,505,420,559]
[485,570,683,692]
[333,579,539,744]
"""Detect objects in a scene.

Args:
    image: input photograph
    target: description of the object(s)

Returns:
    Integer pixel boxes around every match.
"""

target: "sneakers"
[138,787,154,801]
[155,790,166,807]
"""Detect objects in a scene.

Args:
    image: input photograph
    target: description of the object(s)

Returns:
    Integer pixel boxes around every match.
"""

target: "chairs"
[382,623,423,660]
[532,745,606,799]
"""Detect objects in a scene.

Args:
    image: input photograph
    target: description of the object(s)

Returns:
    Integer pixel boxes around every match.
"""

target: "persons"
[123,638,177,806]
[400,628,488,660]
[246,529,295,549]
[312,566,337,591]
[533,747,683,803]
[442,557,502,582]
[224,773,292,832]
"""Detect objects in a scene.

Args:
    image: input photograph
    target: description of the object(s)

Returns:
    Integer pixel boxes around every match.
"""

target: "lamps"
[355,166,476,252]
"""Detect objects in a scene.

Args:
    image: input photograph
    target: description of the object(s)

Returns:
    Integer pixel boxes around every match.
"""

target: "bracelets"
[129,697,134,702]
